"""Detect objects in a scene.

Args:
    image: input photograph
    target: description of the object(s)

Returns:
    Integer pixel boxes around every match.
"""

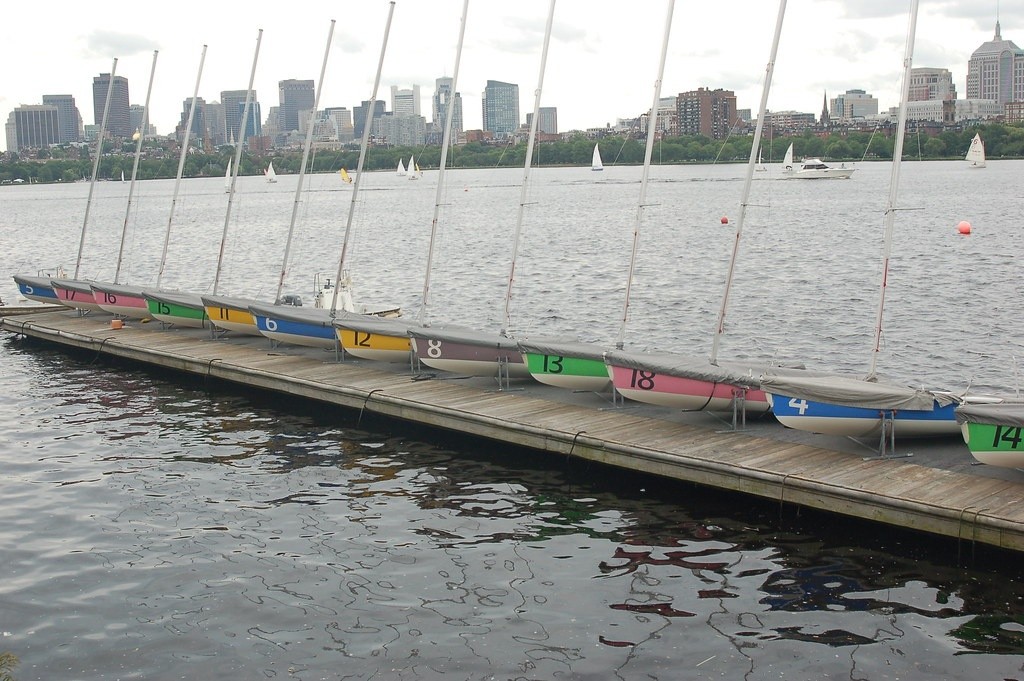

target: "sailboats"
[340,168,352,186]
[262,160,279,184]
[406,155,419,181]
[396,157,406,176]
[10,2,1024,472]
[755,146,767,171]
[795,159,855,179]
[781,141,796,173]
[591,141,604,171]
[222,154,239,195]
[963,131,990,171]
